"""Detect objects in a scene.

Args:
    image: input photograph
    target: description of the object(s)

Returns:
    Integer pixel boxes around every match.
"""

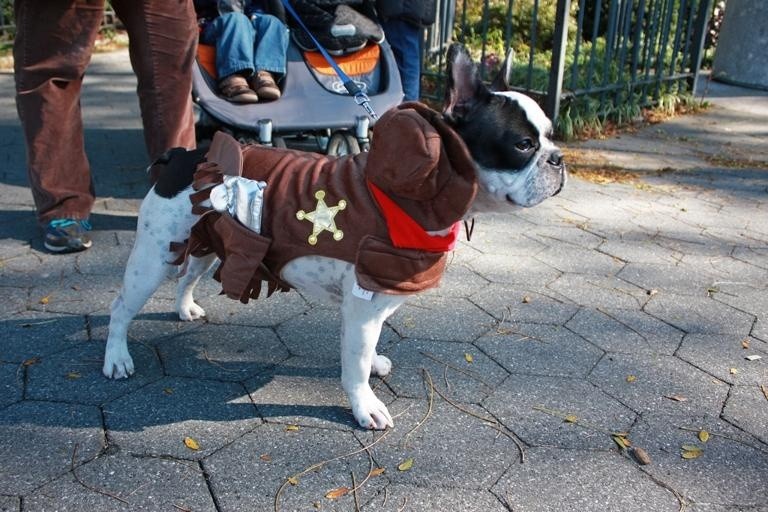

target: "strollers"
[190,3,405,159]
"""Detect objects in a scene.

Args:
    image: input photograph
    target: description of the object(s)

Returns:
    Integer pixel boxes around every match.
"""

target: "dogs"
[102,43,566,433]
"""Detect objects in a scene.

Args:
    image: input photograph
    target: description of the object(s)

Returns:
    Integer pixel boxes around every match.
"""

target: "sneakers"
[219,71,281,104]
[45,218,93,252]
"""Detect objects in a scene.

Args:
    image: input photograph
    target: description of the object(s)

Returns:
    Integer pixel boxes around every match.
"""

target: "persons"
[195,0,290,103]
[12,0,200,253]
[378,0,438,103]
